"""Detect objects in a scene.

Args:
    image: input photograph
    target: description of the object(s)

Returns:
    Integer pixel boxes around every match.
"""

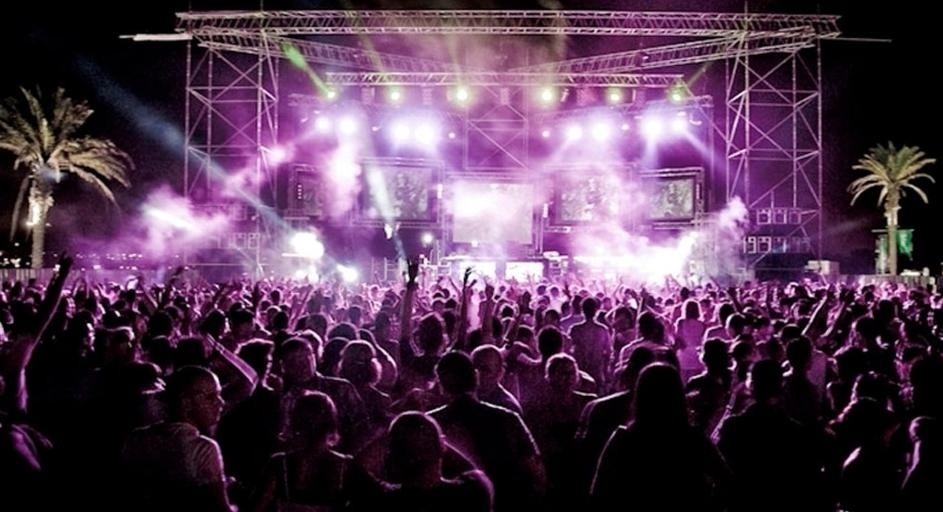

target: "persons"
[586,177,608,219]
[663,182,685,219]
[2,249,942,512]
[394,171,416,218]
